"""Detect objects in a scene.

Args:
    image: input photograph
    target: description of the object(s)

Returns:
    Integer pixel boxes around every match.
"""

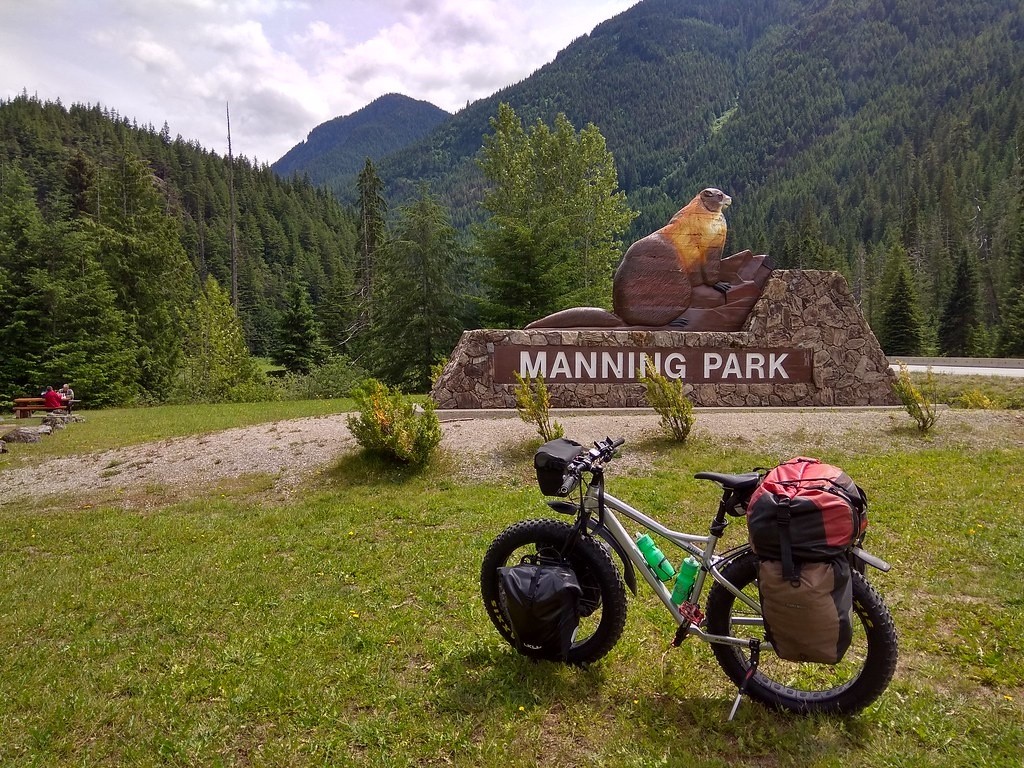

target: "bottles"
[670,555,699,605]
[635,531,676,582]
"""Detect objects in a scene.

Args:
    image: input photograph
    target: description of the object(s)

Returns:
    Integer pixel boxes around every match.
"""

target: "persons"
[57,384,74,411]
[43,386,71,410]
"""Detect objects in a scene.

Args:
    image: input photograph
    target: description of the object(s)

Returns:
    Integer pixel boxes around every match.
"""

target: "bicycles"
[478,435,898,724]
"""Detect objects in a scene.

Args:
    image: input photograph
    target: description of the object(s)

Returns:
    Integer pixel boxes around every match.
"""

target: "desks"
[13,398,81,407]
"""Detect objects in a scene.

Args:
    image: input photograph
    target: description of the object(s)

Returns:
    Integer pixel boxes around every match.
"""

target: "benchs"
[13,406,67,418]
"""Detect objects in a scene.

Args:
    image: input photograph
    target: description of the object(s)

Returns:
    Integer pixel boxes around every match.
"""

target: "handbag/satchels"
[725,456,868,560]
[753,556,853,665]
[496,537,604,663]
